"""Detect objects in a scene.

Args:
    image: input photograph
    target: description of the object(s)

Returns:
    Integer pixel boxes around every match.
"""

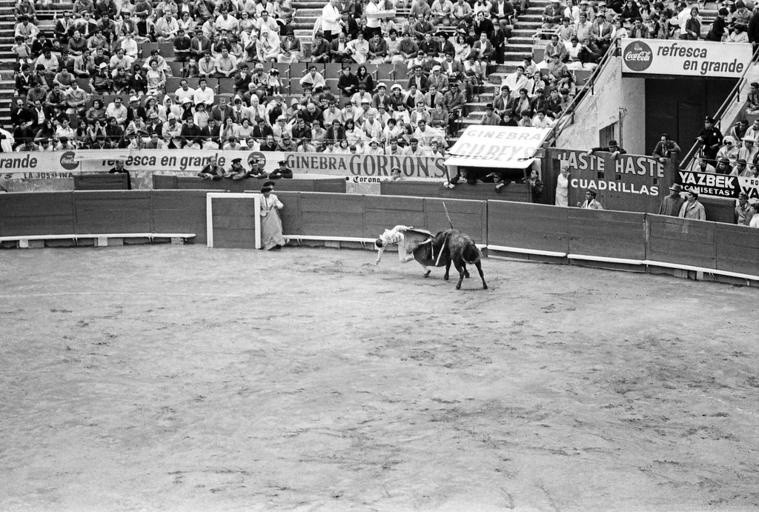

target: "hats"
[128,96,206,120]
[368,138,380,145]
[250,159,259,165]
[280,131,291,139]
[705,117,715,123]
[688,189,699,196]
[277,115,287,122]
[260,182,275,193]
[578,2,606,17]
[231,158,242,165]
[279,161,288,166]
[670,184,682,193]
[741,136,757,143]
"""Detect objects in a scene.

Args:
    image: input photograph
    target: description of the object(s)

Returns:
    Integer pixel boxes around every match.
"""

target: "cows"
[419,229,488,289]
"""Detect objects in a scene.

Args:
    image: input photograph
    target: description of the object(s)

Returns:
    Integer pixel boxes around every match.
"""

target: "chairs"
[79,2,600,134]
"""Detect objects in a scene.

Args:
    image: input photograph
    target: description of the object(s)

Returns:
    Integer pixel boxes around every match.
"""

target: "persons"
[260,187,288,250]
[375,224,416,263]
